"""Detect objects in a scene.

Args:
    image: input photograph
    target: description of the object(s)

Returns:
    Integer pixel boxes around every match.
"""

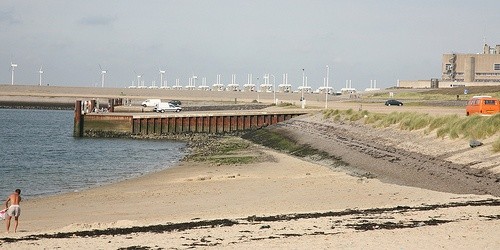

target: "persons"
[5,189,22,233]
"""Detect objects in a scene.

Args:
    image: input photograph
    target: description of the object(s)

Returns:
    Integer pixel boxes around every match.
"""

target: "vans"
[140,99,162,107]
[156,102,182,112]
[464,96,500,115]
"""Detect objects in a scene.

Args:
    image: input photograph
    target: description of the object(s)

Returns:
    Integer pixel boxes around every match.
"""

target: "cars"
[386,100,403,106]
[168,99,181,106]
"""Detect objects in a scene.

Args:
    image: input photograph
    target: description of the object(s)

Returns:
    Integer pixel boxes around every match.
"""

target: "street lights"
[255,64,330,110]
